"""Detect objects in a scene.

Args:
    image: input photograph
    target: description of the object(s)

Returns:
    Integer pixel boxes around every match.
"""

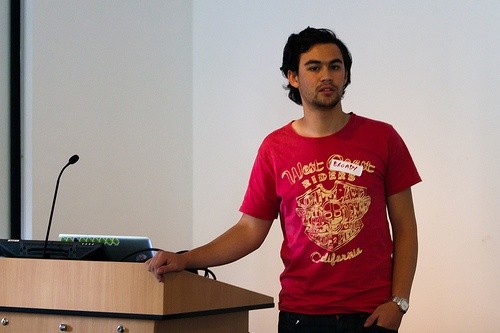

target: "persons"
[145,27,422,333]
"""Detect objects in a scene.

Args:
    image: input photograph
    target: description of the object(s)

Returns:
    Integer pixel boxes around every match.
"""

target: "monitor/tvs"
[0,234,154,263]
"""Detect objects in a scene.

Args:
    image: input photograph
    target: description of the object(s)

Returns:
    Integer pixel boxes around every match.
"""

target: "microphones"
[42,155,80,258]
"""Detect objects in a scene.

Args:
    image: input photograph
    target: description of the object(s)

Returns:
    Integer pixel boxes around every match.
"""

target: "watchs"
[389,296,409,314]
